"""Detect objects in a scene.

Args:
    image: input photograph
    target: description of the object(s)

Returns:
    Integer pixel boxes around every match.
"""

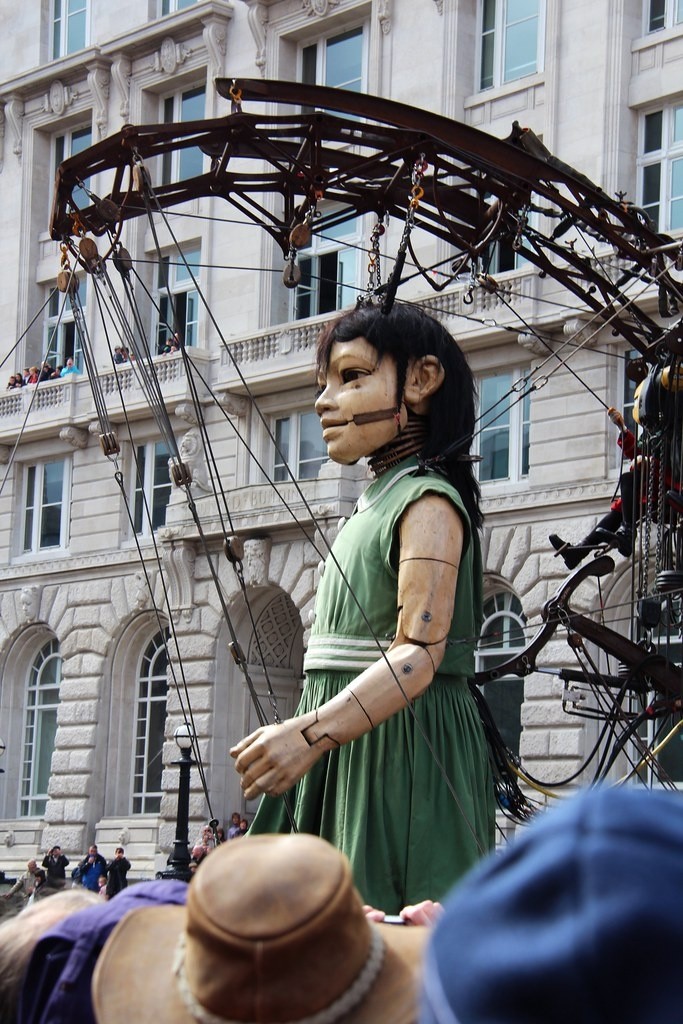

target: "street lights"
[158,722,202,883]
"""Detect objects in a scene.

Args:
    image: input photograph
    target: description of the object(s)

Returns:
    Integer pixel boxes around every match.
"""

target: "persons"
[19,879,189,1023]
[89,833,448,1024]
[97,874,113,901]
[42,846,69,887]
[548,408,683,569]
[0,888,105,1024]
[105,847,131,899]
[26,869,46,898]
[230,301,497,914]
[217,828,225,845]
[7,332,182,387]
[3,858,40,899]
[418,788,683,1023]
[231,819,248,838]
[196,824,215,851]
[0,870,28,918]
[79,845,107,892]
[190,844,206,874]
[227,813,240,839]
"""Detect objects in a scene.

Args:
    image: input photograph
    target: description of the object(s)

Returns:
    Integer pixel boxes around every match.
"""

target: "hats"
[417,781,683,1024]
[93,833,422,1024]
